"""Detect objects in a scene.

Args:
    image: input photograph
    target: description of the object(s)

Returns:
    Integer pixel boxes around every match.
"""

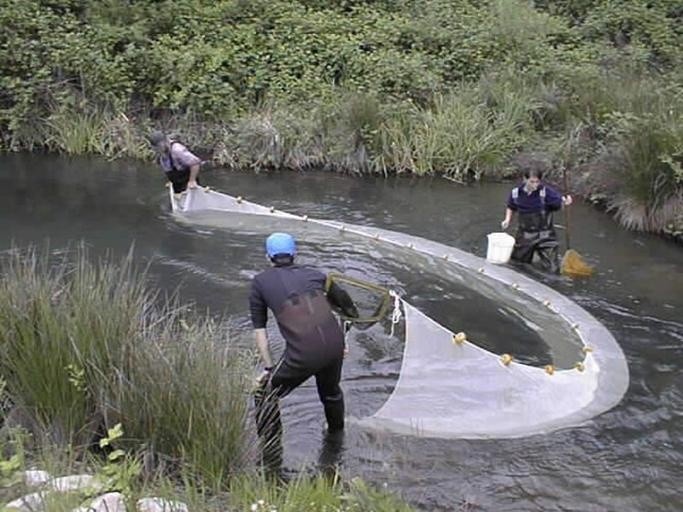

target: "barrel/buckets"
[487,221,516,265]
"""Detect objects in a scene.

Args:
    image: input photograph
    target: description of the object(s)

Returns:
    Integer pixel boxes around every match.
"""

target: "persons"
[498,166,573,278]
[245,229,360,477]
[145,130,203,212]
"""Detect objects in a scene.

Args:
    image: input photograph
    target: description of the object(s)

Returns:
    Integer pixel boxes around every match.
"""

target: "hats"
[264,232,295,259]
